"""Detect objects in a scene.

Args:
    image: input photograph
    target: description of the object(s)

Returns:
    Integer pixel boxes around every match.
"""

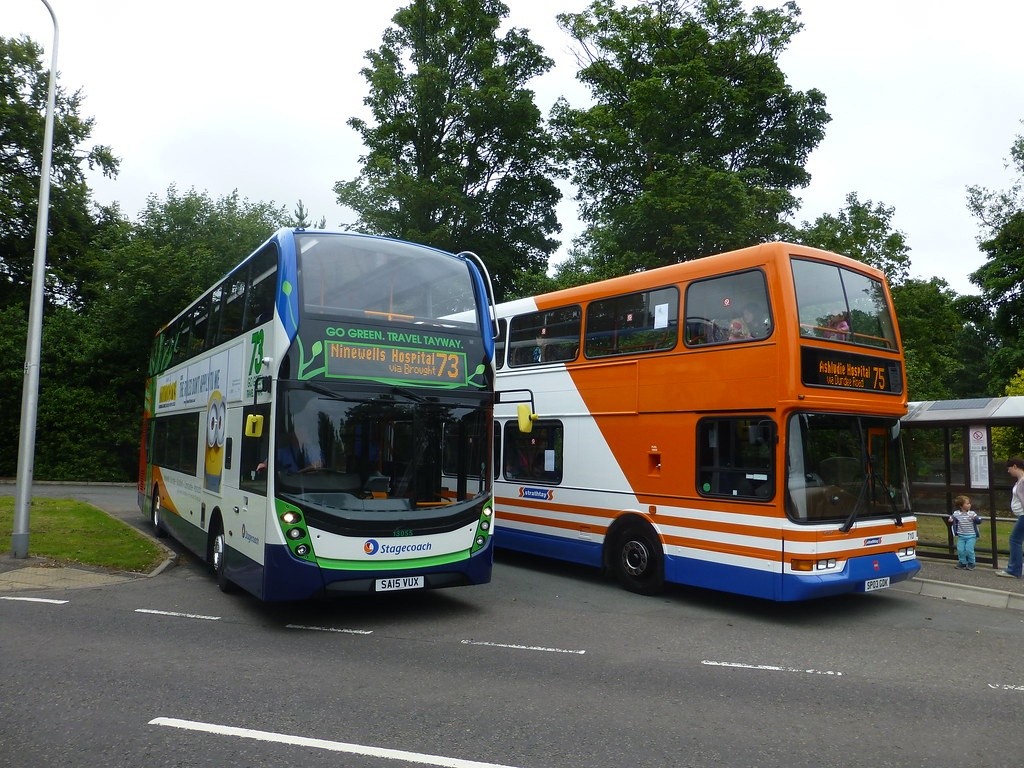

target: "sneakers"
[954,562,966,569]
[966,563,976,570]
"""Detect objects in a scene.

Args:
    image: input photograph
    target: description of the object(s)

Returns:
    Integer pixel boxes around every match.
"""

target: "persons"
[728,303,849,341]
[532,333,573,363]
[256,425,326,478]
[995,456,1024,579]
[948,495,982,571]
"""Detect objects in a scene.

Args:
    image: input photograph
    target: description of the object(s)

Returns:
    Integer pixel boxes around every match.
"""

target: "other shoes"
[995,570,1018,578]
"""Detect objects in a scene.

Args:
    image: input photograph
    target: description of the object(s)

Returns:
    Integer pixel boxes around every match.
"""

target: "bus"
[136,227,538,612]
[410,243,917,602]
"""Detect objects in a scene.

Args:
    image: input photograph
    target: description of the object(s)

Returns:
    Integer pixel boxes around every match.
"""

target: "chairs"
[496,344,562,368]
[707,319,729,343]
